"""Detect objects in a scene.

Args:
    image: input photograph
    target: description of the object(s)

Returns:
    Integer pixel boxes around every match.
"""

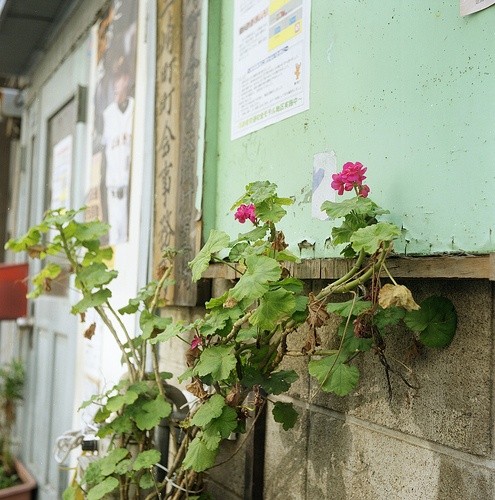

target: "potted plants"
[0,358,37,500]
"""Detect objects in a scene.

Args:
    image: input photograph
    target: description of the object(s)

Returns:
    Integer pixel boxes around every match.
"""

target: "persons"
[82,29,135,244]
